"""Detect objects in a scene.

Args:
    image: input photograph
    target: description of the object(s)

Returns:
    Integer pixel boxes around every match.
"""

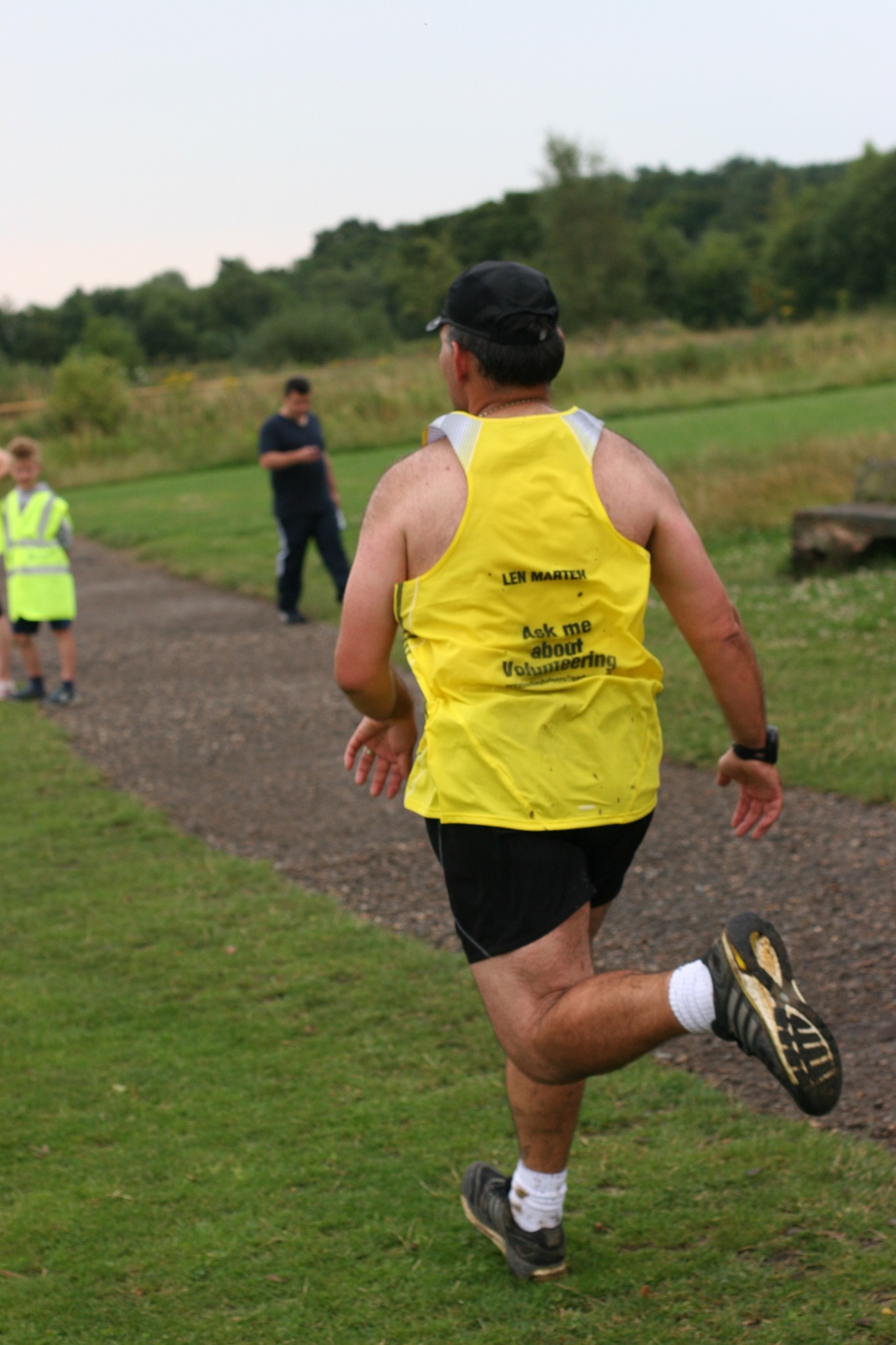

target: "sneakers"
[700,911,843,1116]
[458,1162,567,1284]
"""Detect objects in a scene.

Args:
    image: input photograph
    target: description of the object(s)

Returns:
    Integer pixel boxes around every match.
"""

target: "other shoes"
[279,610,306,624]
[46,687,75,704]
[13,679,43,699]
[1,682,12,699]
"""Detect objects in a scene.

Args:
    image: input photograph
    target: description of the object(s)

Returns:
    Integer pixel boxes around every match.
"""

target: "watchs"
[731,724,781,765]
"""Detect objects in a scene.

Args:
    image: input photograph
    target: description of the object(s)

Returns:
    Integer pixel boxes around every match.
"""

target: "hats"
[425,263,559,346]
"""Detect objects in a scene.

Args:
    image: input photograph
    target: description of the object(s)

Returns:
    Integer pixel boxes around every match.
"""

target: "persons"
[0,434,81,709]
[2,442,22,705]
[255,375,359,628]
[333,248,848,1281]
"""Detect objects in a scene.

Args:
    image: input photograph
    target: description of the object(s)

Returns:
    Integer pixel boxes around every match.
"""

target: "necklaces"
[475,394,554,418]
